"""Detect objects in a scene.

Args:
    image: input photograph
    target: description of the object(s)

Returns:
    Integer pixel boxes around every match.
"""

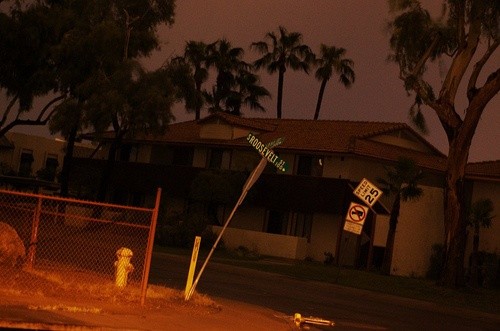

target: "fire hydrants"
[113,247,135,287]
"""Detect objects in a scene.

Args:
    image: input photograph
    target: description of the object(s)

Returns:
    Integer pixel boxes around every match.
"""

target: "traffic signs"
[246,132,289,173]
[352,177,382,207]
